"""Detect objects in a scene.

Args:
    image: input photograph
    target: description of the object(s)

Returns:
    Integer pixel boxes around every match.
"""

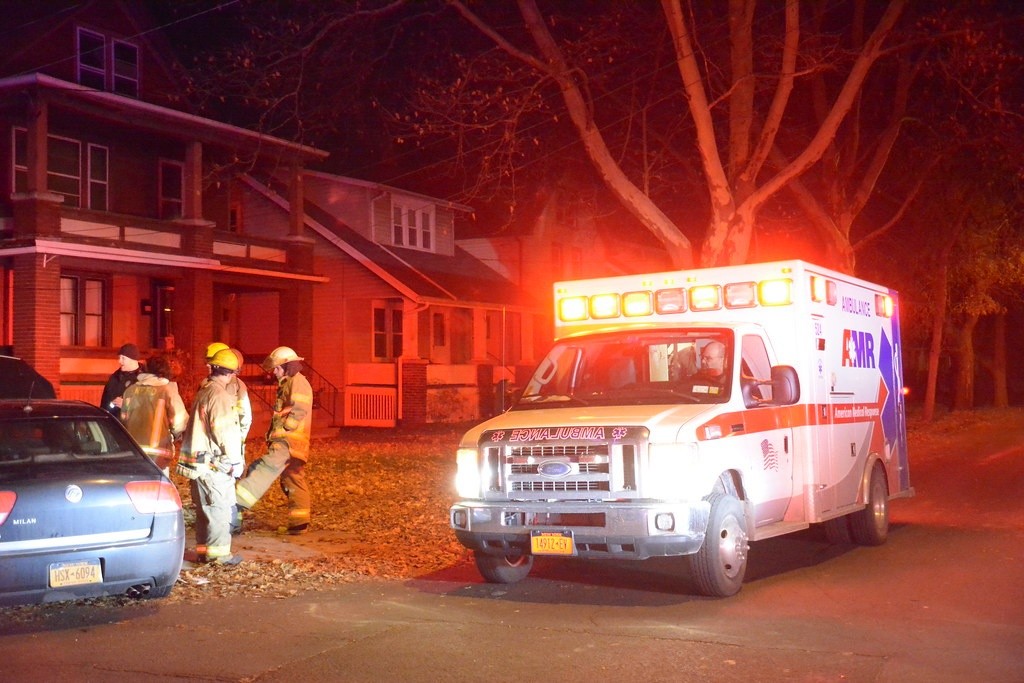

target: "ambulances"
[451,256,917,602]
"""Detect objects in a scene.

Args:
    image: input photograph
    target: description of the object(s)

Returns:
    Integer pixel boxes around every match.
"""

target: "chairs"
[44,422,81,455]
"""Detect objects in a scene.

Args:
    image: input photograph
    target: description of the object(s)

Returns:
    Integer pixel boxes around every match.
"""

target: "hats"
[117,343,140,360]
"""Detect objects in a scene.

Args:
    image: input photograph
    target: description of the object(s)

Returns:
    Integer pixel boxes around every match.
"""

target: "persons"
[99,342,312,568]
[690,340,729,387]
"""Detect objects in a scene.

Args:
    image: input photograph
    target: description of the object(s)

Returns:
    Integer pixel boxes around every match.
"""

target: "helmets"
[201,343,244,371]
[263,346,305,371]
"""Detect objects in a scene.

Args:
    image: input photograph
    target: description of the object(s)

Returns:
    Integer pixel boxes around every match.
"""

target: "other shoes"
[198,555,243,566]
[288,524,308,534]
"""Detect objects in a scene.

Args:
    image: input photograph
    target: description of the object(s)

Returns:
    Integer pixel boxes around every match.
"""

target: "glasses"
[699,354,724,362]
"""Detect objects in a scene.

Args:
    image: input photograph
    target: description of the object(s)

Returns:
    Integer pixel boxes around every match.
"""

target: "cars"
[0,396,186,607]
